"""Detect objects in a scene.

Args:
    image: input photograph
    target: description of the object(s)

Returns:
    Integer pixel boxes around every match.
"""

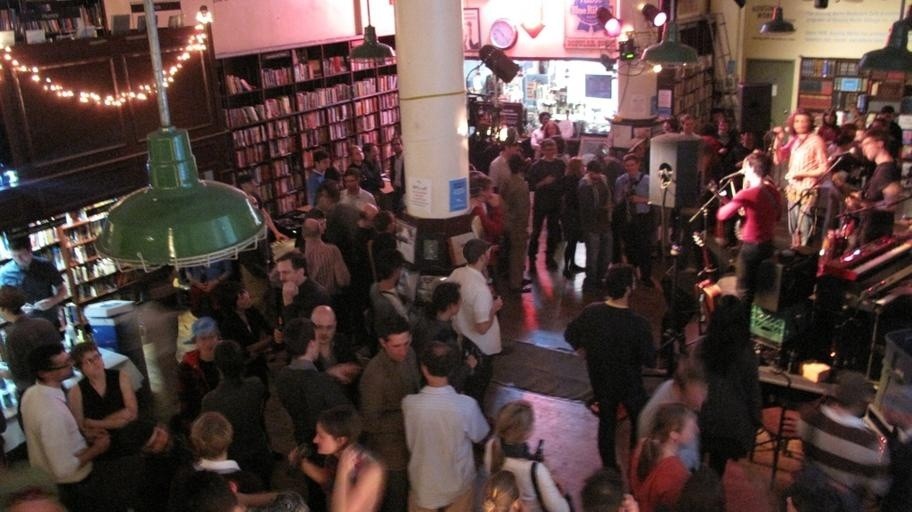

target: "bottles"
[273,316,284,350]
[787,348,799,373]
[463,348,476,379]
[533,439,545,461]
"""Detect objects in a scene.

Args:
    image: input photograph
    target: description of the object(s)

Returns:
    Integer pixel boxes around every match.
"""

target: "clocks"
[489,19,518,51]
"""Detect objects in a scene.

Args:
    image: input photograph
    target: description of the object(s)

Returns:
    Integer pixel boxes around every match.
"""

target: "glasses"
[81,354,101,368]
[46,354,74,370]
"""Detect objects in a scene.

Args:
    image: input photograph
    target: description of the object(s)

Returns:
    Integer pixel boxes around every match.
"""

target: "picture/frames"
[463,7,481,53]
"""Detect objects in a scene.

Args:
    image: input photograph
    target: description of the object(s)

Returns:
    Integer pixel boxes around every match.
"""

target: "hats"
[183,317,219,344]
[214,340,246,375]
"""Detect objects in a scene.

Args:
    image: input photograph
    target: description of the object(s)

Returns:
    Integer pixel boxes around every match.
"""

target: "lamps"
[642,4,667,39]
[761,0,797,36]
[857,0,912,74]
[347,1,394,59]
[598,7,623,35]
[466,44,520,85]
[92,0,268,273]
[640,0,698,66]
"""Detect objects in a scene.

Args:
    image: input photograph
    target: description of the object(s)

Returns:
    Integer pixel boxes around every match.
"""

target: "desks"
[749,364,837,492]
[0,346,144,468]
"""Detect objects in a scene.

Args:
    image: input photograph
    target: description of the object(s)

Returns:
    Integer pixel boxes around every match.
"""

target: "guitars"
[831,173,856,229]
[691,232,722,327]
[706,181,746,240]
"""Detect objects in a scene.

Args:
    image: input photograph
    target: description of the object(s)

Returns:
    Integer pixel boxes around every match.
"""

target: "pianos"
[814,231,912,314]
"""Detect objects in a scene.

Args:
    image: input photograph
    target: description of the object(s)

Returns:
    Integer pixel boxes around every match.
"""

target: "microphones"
[771,127,790,134]
[831,147,856,158]
[721,168,745,178]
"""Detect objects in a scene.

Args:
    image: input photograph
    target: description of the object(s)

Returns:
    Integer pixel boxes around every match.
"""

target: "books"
[798,54,911,191]
[657,54,713,126]
[216,37,399,223]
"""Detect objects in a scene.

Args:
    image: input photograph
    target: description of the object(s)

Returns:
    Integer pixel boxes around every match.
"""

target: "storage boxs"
[84,299,143,353]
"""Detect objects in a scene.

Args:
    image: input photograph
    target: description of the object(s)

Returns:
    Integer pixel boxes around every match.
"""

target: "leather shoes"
[510,286,532,292]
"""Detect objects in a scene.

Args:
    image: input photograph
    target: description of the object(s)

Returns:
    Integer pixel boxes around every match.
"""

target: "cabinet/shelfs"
[0,0,111,47]
[1,164,221,340]
[797,57,912,113]
[211,35,402,219]
[655,51,714,132]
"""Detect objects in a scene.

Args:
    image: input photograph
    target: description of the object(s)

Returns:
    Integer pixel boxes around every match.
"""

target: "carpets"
[486,335,672,408]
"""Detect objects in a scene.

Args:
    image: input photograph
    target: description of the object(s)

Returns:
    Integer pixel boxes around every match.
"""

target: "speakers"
[751,246,820,314]
[736,80,772,147]
[646,132,703,211]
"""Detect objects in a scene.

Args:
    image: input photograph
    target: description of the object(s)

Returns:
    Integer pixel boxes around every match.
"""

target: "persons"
[779,370,892,512]
[882,386,911,512]
[483,400,575,510]
[637,358,708,472]
[694,294,764,475]
[668,112,912,326]
[580,467,639,512]
[466,109,657,295]
[1,137,526,511]
[564,264,656,471]
[783,469,844,512]
[676,467,728,512]
[628,403,700,512]
